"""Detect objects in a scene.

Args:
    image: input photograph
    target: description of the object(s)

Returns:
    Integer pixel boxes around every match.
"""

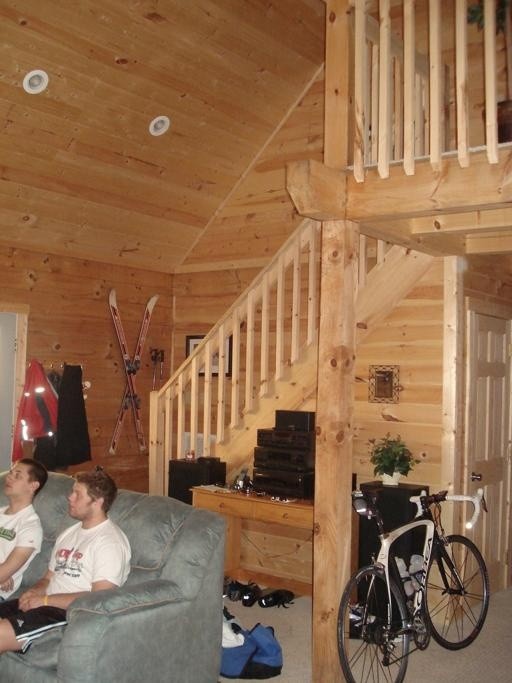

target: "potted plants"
[367,429,423,486]
[464,0,510,141]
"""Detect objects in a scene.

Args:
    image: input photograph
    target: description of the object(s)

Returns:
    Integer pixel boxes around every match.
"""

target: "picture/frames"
[368,363,402,406]
[185,334,234,377]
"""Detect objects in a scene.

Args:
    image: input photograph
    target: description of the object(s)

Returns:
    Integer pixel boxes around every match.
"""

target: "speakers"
[168,458,226,505]
[357,481,429,620]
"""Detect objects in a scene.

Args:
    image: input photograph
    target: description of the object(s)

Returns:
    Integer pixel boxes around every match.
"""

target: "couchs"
[0,464,228,681]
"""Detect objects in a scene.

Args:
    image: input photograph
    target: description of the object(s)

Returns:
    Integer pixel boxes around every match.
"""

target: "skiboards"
[109,287,160,456]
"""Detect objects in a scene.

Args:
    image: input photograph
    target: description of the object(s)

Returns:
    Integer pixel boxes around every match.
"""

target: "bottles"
[230,472,253,494]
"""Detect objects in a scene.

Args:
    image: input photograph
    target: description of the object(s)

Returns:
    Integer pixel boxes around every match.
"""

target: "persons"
[1,457,49,604]
[0,469,133,652]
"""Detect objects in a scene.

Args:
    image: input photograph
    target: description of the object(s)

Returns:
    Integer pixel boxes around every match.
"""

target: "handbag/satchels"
[220,607,284,681]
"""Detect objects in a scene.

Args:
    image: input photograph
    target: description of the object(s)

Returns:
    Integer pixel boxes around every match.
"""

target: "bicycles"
[336,487,489,682]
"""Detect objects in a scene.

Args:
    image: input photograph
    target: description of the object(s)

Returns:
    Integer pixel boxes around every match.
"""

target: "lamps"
[22,68,49,96]
[148,114,172,137]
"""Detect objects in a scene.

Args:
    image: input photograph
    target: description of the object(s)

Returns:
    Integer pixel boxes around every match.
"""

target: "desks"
[188,483,360,606]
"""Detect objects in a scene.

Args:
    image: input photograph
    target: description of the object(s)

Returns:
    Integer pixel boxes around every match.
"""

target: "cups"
[183,449,195,463]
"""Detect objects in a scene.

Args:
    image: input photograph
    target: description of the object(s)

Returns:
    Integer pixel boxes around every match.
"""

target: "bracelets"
[43,595,51,606]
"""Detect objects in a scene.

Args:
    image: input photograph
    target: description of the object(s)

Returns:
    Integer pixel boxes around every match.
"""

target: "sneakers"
[223,577,261,607]
[257,589,295,609]
